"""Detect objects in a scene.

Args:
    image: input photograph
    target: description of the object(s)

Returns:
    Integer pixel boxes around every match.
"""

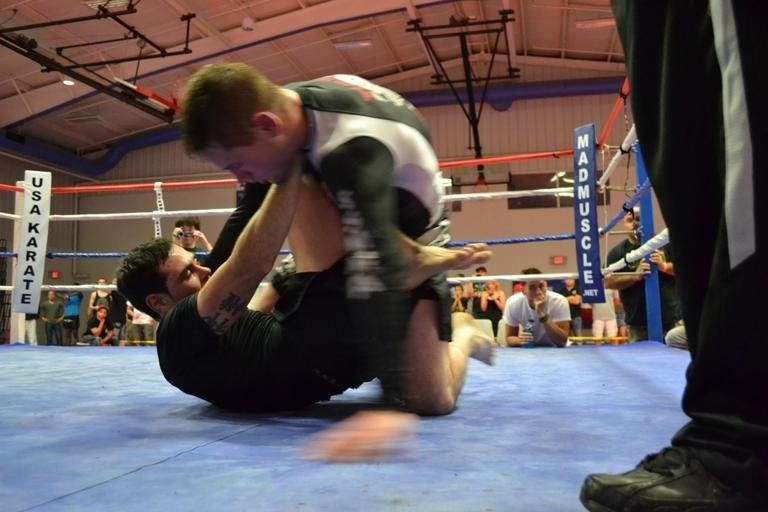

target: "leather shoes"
[579,447,768,512]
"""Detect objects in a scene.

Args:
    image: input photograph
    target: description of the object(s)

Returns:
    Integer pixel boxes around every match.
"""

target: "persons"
[580,2,765,512]
[447,264,696,348]
[178,59,499,415]
[170,214,214,260]
[602,202,679,346]
[40,276,158,347]
[115,152,494,414]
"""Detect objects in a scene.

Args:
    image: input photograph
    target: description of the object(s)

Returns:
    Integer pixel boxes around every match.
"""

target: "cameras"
[178,231,193,238]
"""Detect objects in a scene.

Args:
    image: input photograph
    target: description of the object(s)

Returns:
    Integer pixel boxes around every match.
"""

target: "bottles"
[521,319,535,349]
